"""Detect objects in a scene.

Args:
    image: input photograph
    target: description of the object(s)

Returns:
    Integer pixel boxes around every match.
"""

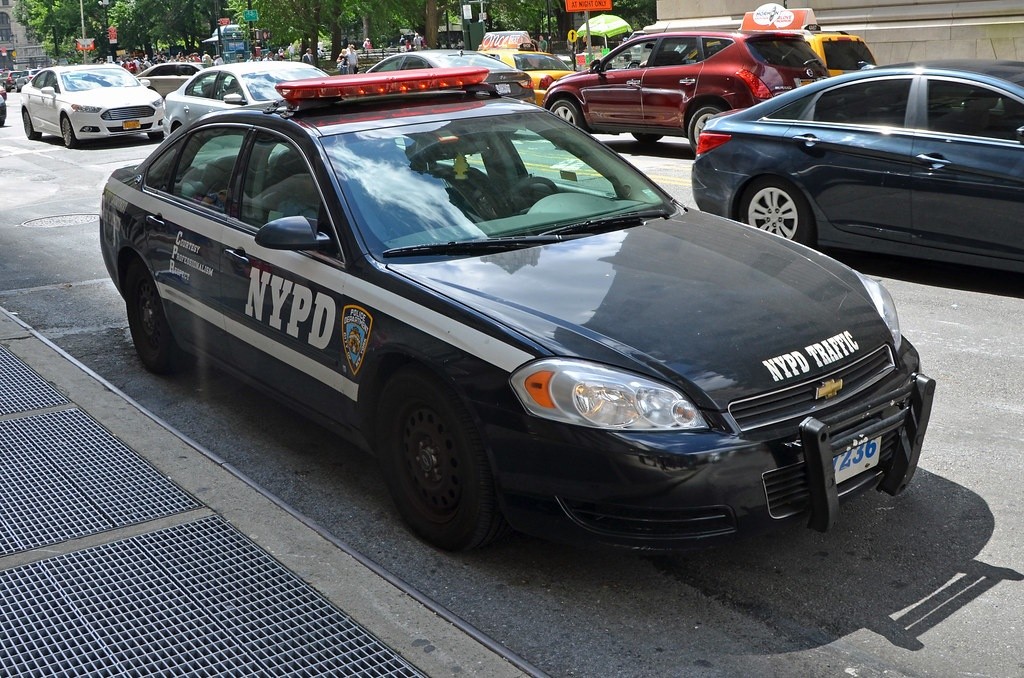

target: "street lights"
[10,34,16,60]
[98,0,110,55]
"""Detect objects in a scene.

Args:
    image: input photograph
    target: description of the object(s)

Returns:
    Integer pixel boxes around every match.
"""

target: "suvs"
[541,31,833,156]
[0,71,23,92]
[14,69,41,93]
[687,7,877,87]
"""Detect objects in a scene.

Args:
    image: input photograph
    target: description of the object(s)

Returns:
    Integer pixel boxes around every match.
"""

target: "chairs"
[182,145,512,225]
[651,50,681,65]
[202,79,221,98]
[963,94,991,137]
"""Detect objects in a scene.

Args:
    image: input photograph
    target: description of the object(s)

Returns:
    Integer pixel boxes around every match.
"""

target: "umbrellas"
[576,14,634,51]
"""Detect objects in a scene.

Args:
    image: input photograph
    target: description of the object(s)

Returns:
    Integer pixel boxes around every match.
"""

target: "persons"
[436,37,465,49]
[95,49,224,76]
[566,39,576,61]
[26,66,29,70]
[336,44,359,74]
[37,65,42,69]
[390,32,424,52]
[362,38,372,54]
[248,40,326,65]
[201,189,227,213]
[530,34,547,53]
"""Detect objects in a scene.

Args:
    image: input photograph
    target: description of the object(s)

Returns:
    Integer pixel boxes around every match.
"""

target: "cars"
[0,85,7,101]
[162,61,331,140]
[21,64,166,149]
[100,69,936,559]
[693,60,1024,273]
[134,62,205,98]
[476,49,578,106]
[365,49,536,105]
[0,94,7,127]
